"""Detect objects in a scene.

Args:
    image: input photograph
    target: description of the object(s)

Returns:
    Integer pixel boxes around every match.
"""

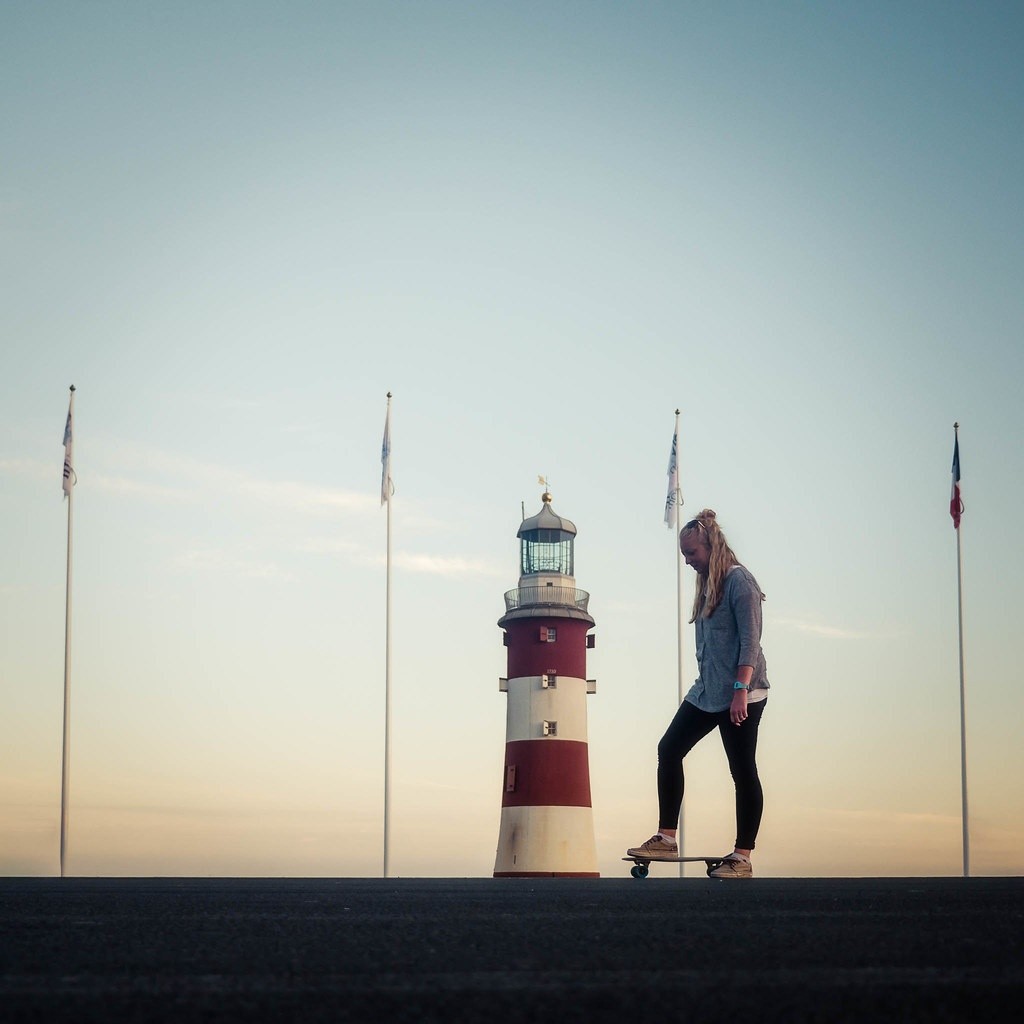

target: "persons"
[627,511,768,878]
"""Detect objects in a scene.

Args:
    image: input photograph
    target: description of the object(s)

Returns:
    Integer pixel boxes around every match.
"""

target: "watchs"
[734,682,750,690]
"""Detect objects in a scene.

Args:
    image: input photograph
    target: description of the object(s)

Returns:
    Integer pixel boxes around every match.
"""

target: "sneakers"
[711,857,754,878]
[626,836,678,858]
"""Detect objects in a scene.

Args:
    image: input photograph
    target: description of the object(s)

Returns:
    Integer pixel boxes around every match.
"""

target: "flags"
[664,426,679,529]
[62,403,71,495]
[381,405,392,506]
[950,430,962,531]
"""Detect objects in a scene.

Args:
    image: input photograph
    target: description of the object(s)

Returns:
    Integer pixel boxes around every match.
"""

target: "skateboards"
[621,856,741,878]
